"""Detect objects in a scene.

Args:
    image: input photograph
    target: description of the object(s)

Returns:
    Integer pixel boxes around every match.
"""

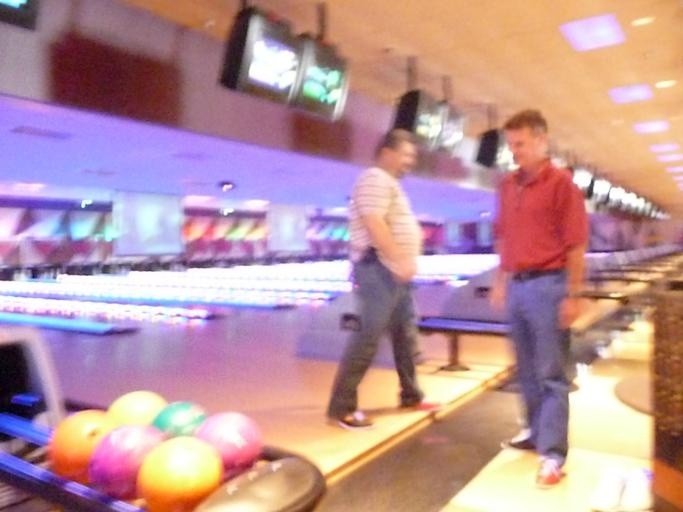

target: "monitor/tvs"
[476,130,671,221]
[1,0,41,30]
[434,99,468,154]
[219,7,307,105]
[393,89,446,150]
[289,31,352,123]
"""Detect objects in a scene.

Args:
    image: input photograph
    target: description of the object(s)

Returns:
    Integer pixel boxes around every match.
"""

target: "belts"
[513,270,554,281]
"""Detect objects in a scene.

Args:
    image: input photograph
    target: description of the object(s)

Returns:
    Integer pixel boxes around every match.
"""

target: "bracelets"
[566,291,583,297]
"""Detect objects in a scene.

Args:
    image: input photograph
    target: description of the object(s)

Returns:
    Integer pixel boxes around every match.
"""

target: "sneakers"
[402,395,443,413]
[499,439,535,452]
[536,460,562,488]
[337,410,374,430]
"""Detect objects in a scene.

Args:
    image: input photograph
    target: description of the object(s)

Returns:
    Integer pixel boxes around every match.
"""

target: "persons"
[326,129,439,431]
[488,108,588,485]
